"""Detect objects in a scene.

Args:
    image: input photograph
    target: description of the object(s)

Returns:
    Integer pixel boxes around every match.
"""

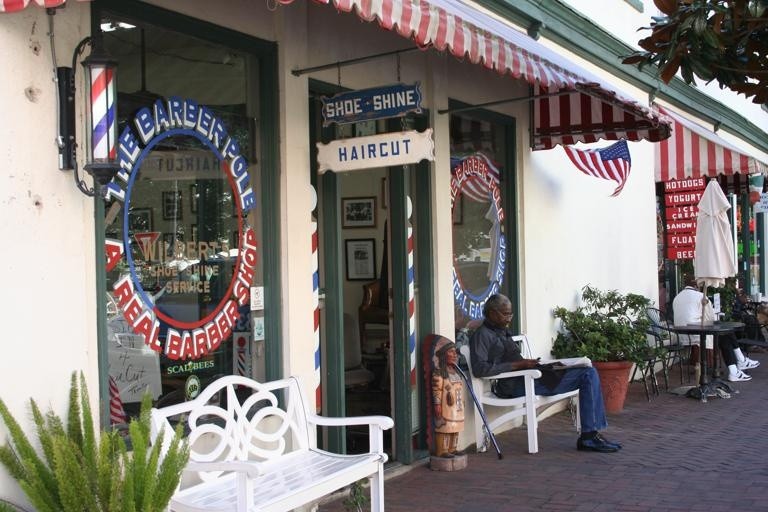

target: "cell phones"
[537,358,540,360]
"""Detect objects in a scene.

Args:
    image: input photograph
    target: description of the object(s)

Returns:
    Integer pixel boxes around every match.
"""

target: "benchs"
[461,336,591,454]
[148,375,394,512]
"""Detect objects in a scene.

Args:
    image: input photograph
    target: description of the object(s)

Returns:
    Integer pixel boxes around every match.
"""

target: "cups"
[752,292,762,303]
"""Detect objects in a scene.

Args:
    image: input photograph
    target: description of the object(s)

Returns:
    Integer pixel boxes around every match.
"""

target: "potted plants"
[551,284,667,412]
[691,272,745,368]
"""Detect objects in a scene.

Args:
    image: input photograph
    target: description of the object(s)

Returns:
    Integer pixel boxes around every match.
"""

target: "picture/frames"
[163,233,182,242]
[191,223,197,242]
[382,177,388,209]
[452,192,463,224]
[340,196,377,228]
[352,120,378,136]
[233,231,239,247]
[232,188,248,219]
[190,184,197,212]
[129,207,153,244]
[345,238,377,281]
[162,191,183,219]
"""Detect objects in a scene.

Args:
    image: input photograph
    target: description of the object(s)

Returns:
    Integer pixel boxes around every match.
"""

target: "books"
[550,356,593,372]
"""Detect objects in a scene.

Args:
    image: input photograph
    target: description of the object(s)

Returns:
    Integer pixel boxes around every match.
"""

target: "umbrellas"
[692,176,738,327]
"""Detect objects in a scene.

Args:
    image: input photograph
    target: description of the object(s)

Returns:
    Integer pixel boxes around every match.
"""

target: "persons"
[671,273,761,382]
[719,279,766,352]
[470,292,625,453]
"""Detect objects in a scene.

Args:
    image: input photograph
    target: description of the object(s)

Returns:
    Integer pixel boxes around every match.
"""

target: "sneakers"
[738,356,760,370]
[728,370,752,382]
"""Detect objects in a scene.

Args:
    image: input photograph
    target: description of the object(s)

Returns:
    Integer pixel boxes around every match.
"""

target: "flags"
[561,139,633,198]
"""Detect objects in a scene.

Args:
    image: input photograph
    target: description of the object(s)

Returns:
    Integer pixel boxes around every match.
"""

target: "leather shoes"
[576,432,624,451]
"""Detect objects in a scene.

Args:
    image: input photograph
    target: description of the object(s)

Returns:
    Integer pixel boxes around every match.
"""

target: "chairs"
[733,302,768,352]
[632,320,669,402]
[646,308,691,385]
[343,311,375,393]
